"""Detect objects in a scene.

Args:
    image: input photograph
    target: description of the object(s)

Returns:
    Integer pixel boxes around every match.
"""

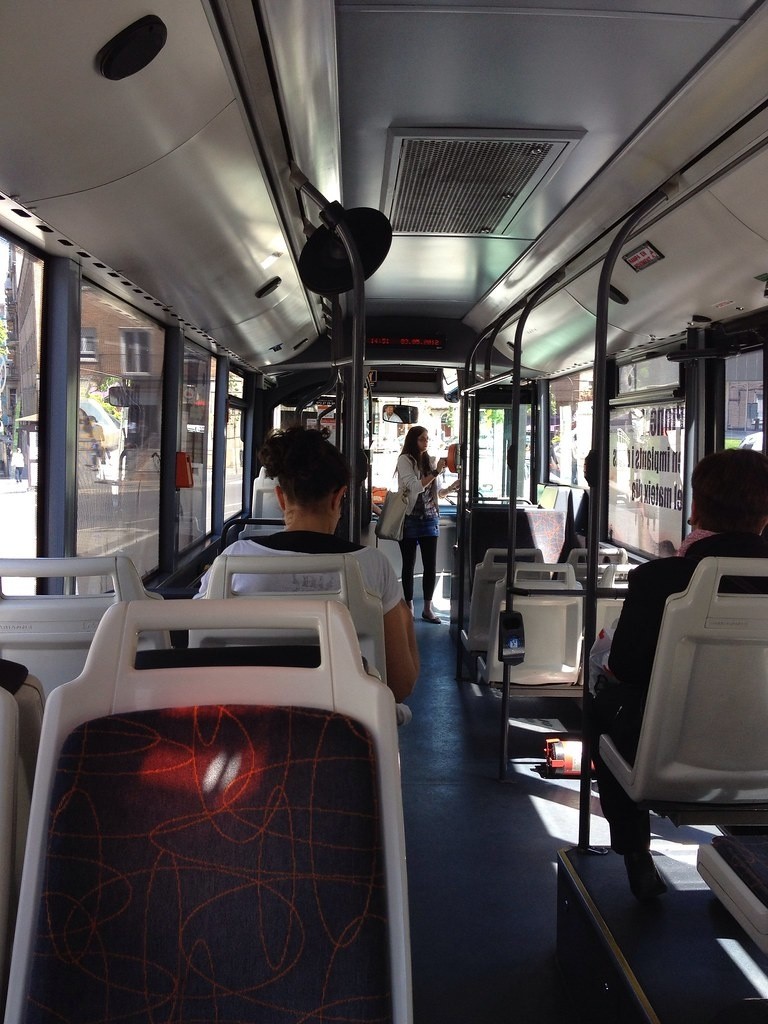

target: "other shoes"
[396,702,412,728]
[626,848,668,900]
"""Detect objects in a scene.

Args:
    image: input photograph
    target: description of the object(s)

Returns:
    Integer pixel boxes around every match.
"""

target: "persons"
[372,503,381,516]
[397,426,459,623]
[11,448,24,483]
[88,415,111,478]
[199,423,420,725]
[384,406,402,422]
[594,448,768,899]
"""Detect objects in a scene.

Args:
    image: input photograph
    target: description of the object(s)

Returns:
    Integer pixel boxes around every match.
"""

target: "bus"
[109,379,243,547]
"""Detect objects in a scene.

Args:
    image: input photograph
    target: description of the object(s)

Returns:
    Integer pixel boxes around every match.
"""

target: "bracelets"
[447,486,455,494]
[432,470,439,477]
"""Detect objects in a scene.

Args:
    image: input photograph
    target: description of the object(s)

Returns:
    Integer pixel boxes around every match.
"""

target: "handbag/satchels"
[375,454,414,540]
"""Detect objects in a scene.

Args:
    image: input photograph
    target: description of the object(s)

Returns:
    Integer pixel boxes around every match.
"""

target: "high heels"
[422,611,441,624]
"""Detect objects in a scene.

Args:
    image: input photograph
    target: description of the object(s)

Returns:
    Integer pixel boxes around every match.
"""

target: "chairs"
[0,463,413,1024]
[451,488,768,954]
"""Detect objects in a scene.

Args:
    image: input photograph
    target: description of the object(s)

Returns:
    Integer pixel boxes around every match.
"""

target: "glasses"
[418,438,428,442]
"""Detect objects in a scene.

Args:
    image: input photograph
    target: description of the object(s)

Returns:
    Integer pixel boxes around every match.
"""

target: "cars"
[364,397,763,520]
